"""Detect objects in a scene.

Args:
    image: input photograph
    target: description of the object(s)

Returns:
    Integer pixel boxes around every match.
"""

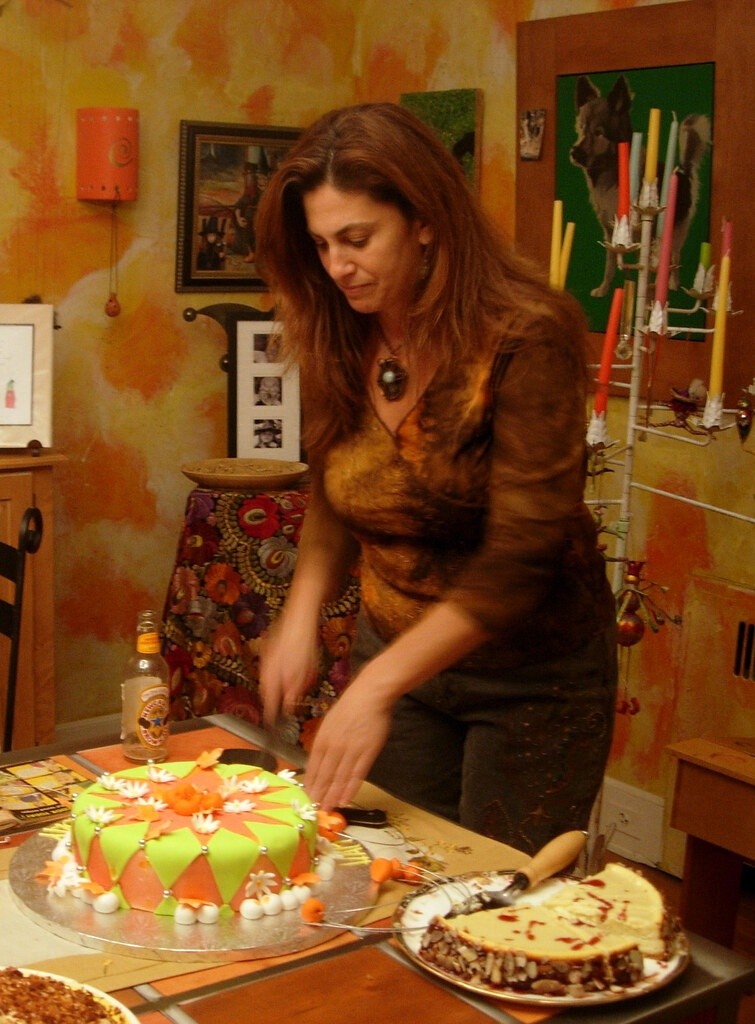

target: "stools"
[663,739,755,949]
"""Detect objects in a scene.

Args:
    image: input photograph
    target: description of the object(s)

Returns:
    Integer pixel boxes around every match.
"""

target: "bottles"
[122,610,168,764]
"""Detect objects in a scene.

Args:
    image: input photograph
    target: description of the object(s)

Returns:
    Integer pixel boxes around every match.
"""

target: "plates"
[393,870,689,1007]
[181,458,311,491]
[0,966,140,1024]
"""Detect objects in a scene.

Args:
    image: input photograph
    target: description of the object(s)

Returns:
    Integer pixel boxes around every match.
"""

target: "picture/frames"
[226,312,307,463]
[176,120,306,295]
[0,303,53,449]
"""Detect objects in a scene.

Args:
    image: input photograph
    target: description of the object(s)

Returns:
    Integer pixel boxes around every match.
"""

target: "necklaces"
[372,324,411,403]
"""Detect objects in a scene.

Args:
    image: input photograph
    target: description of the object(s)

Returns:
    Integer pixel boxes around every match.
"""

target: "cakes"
[34,747,347,924]
[416,859,680,997]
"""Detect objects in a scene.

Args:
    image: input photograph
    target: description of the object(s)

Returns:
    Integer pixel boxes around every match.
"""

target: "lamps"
[75,107,139,202]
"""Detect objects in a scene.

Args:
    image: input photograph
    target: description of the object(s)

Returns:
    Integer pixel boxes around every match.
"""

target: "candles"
[630,132,643,209]
[560,223,576,288]
[654,165,680,308]
[657,110,678,241]
[645,106,661,183]
[616,141,630,227]
[548,200,563,290]
[723,220,734,254]
[710,253,730,399]
[701,243,712,272]
[592,287,625,419]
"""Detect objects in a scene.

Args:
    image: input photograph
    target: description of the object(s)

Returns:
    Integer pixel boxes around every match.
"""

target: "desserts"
[0,967,128,1024]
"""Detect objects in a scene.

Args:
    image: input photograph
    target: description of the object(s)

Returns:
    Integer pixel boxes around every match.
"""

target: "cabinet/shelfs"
[0,449,68,753]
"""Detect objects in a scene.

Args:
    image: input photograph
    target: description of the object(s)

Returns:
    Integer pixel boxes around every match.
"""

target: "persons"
[254,421,283,448]
[255,104,618,879]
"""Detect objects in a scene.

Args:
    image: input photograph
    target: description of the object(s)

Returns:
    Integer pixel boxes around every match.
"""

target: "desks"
[0,710,755,1024]
[157,472,361,752]
